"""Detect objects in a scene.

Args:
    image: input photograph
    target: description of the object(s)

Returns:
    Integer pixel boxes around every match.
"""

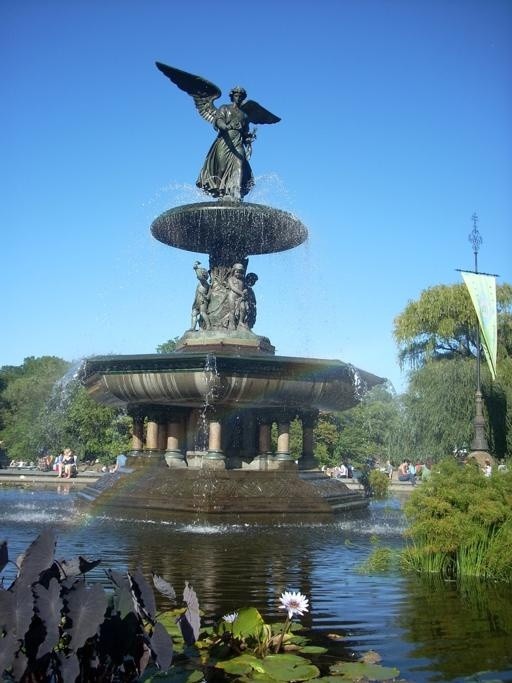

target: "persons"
[188,260,213,330]
[225,260,251,331]
[8,443,115,480]
[321,451,510,486]
[111,449,128,473]
[194,83,259,202]
[243,272,259,330]
